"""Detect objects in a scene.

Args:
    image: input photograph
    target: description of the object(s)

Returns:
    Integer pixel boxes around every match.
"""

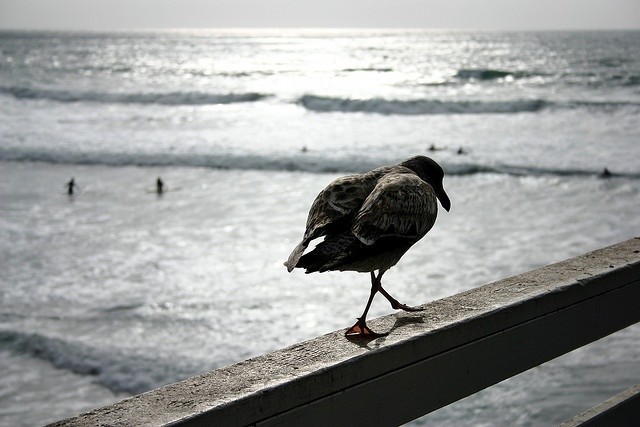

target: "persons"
[156,177,164,192]
[67,179,75,195]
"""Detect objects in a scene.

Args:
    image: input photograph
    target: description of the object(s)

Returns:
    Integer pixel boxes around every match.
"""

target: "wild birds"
[283,152,452,338]
